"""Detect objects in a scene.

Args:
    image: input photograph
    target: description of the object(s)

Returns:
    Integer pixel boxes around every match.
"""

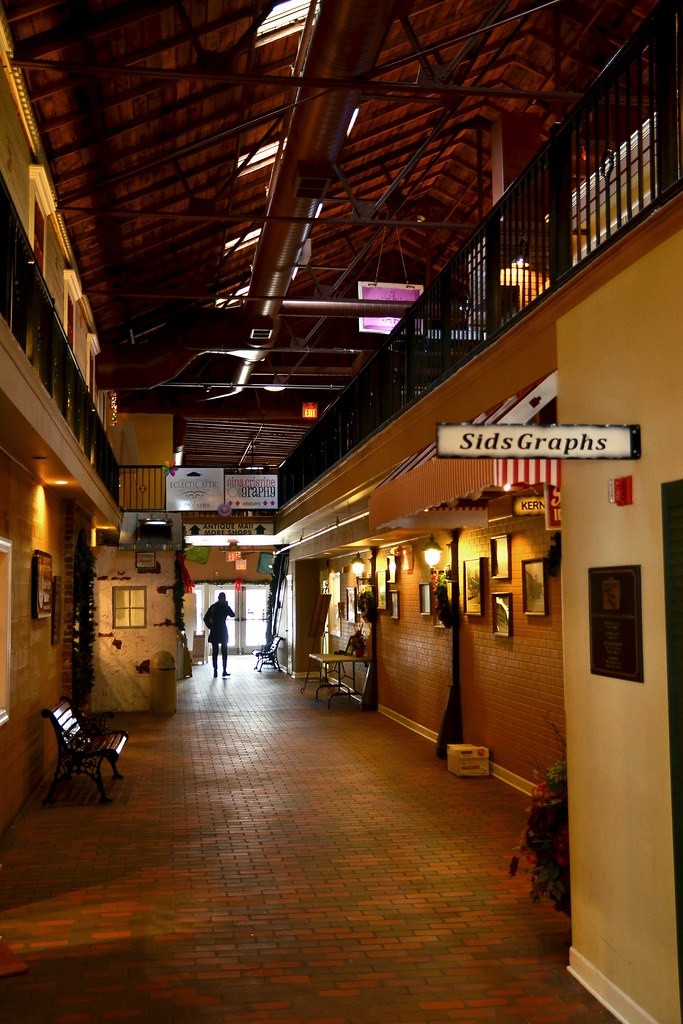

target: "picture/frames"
[388,589,399,619]
[419,582,431,616]
[522,555,546,615]
[491,592,513,637]
[346,586,356,623]
[136,552,155,568]
[375,570,386,610]
[489,534,510,579]
[463,557,484,616]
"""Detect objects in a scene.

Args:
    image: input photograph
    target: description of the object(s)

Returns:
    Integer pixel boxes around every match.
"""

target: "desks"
[309,653,366,708]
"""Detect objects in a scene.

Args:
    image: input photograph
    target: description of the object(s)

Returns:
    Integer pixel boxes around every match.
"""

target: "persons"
[204,592,235,676]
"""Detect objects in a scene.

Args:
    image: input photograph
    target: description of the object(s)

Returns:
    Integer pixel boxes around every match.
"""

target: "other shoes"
[214,671,218,677]
[223,671,230,676]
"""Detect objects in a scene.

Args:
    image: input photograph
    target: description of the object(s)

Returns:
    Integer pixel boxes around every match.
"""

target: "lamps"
[351,553,366,577]
[423,534,444,575]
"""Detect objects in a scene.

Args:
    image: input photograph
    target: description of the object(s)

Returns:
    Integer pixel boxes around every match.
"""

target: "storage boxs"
[447,744,490,777]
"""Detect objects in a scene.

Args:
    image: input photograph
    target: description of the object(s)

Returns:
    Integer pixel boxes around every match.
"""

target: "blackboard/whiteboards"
[192,635,208,661]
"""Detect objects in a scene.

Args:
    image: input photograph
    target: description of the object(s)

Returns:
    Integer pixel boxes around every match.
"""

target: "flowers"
[430,573,453,628]
[507,731,572,915]
[358,590,377,622]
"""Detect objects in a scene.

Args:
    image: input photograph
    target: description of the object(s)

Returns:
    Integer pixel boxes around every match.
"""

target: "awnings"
[369,453,559,529]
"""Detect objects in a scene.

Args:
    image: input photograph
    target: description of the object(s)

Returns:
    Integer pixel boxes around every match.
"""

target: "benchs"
[252,636,282,672]
[41,696,128,805]
